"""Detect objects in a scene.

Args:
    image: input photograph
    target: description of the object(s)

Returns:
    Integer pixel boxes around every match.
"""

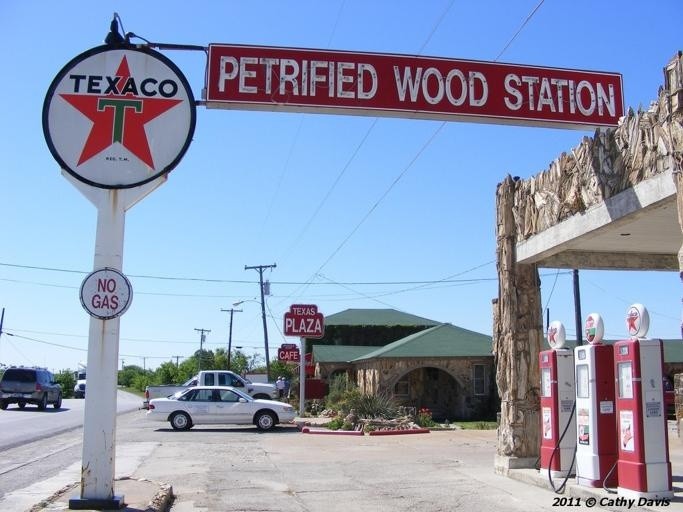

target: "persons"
[276,376,285,401]
[280,377,289,403]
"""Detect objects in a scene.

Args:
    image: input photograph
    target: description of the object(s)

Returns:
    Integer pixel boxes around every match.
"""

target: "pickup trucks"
[144,369,277,404]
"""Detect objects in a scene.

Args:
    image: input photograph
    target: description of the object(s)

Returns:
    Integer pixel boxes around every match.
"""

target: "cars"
[0,367,62,411]
[147,385,296,429]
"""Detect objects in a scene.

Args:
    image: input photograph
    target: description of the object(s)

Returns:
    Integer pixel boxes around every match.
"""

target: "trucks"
[73,366,86,398]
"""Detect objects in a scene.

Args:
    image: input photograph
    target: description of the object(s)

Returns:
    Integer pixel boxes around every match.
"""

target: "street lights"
[233,300,270,376]
